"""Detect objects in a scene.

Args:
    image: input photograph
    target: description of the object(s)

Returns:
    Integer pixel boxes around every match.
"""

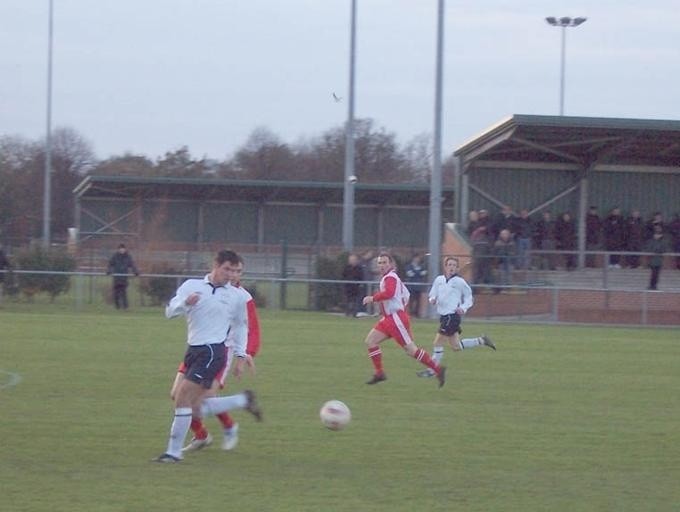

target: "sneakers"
[181,434,213,452]
[483,334,496,350]
[365,372,388,385]
[151,454,184,463]
[222,423,240,451]
[415,370,435,378]
[438,367,446,388]
[245,389,262,421]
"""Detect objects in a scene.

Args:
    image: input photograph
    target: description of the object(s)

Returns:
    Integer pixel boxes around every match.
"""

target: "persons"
[168,253,260,456]
[147,247,262,465]
[104,242,140,311]
[360,251,449,389]
[415,258,499,379]
[342,254,429,320]
[466,204,680,293]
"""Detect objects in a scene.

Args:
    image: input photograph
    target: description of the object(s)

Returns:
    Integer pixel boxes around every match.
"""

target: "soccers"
[320,400,350,430]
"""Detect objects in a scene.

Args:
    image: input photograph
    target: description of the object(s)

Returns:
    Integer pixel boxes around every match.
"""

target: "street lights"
[546,15,587,115]
